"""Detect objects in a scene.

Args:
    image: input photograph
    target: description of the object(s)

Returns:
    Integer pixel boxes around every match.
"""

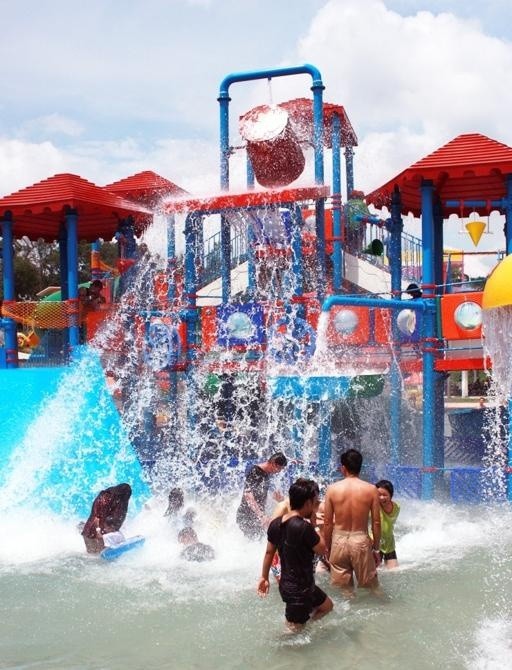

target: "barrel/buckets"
[239,104,305,189]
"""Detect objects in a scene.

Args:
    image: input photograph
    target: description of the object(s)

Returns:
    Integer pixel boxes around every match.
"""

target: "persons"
[408,284,422,299]
[16,322,31,354]
[78,280,107,311]
